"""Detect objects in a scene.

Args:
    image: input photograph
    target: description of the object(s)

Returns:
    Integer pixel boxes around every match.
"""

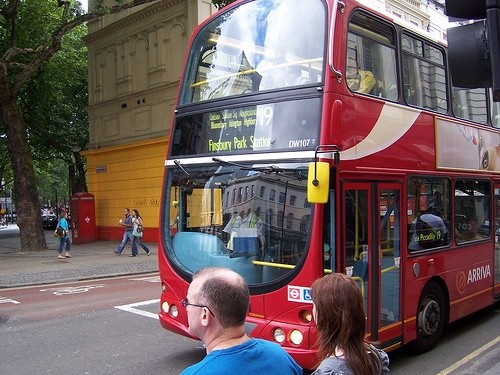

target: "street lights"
[274,178,303,263]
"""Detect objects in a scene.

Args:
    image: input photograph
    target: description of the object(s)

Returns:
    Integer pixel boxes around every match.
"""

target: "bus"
[380,192,448,230]
[156,0,500,372]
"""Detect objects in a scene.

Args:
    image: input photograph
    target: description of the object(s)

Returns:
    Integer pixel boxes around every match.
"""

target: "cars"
[479,220,499,236]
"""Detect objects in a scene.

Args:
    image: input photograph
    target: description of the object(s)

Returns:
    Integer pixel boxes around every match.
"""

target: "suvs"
[42,213,59,229]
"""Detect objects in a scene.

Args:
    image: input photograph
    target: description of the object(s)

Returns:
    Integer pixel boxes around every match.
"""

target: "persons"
[177,266,303,375]
[129,209,152,257]
[346,47,378,94]
[113,208,139,256]
[307,272,390,375]
[222,201,266,256]
[281,66,310,86]
[54,211,71,259]
[407,200,448,253]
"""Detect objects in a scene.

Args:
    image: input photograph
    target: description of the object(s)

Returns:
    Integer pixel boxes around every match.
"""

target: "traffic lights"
[444,0,500,102]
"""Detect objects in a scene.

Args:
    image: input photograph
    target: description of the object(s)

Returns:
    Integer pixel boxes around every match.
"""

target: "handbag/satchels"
[417,215,440,248]
[137,227,144,232]
[56,226,67,239]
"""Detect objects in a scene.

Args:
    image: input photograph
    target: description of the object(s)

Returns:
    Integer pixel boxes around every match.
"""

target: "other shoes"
[58,256,65,259]
[64,255,72,258]
[128,255,136,257]
[147,250,151,257]
[114,251,120,256]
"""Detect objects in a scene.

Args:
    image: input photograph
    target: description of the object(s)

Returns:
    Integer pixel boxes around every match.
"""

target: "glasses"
[181,298,215,317]
